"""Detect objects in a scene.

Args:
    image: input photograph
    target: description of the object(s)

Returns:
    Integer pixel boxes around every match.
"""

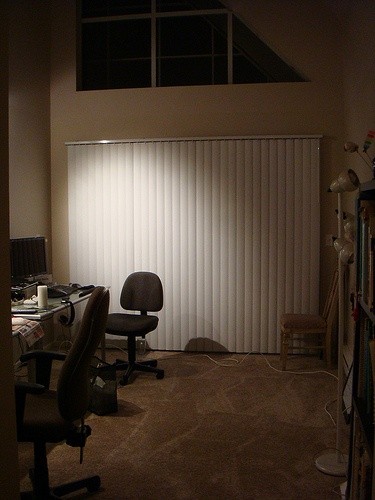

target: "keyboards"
[48,284,75,294]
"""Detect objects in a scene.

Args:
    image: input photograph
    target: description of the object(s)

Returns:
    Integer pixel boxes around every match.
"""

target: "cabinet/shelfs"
[345,180,374,500]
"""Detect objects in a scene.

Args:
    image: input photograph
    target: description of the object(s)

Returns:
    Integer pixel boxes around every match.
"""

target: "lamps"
[315,169,360,477]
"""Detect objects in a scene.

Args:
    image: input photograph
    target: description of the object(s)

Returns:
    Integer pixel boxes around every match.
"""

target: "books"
[349,203,375,500]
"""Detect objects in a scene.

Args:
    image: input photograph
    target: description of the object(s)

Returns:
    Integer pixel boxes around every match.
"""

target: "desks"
[12,289,92,390]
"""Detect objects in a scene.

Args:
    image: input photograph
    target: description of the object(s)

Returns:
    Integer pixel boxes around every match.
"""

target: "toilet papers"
[37,286,49,307]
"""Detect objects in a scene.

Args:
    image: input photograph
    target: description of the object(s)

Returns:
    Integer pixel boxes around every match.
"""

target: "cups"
[37,285,48,309]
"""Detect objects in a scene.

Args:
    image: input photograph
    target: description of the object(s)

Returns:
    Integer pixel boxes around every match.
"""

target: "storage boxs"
[14,285,36,298]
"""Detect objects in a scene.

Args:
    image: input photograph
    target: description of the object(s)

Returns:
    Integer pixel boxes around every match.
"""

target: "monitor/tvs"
[10,236,47,282]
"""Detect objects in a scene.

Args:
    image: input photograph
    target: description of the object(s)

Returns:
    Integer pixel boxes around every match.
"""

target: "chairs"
[14,285,110,500]
[279,270,338,370]
[104,271,164,385]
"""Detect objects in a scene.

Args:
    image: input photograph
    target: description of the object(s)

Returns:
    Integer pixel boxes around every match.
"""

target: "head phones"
[59,299,75,327]
